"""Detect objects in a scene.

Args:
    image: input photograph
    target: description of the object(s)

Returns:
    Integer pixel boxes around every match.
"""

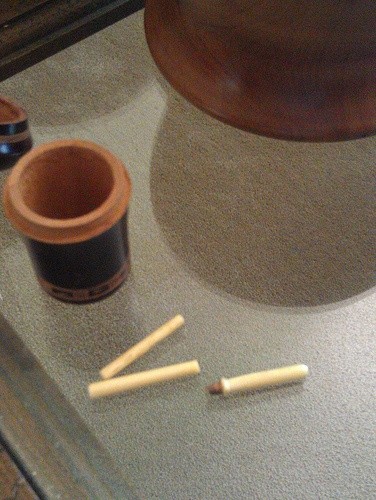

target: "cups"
[5,140,130,303]
